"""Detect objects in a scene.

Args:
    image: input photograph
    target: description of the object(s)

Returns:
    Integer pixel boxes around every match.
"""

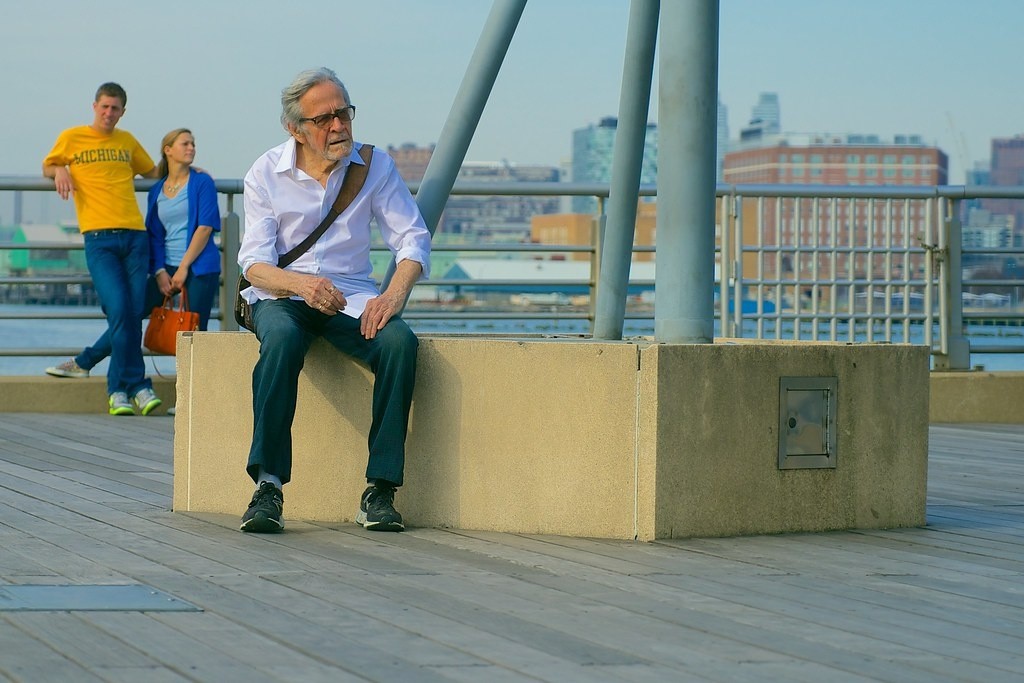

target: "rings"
[322,300,327,305]
[328,286,336,293]
[318,305,323,310]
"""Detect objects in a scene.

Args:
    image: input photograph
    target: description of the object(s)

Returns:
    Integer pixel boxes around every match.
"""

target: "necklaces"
[163,175,189,193]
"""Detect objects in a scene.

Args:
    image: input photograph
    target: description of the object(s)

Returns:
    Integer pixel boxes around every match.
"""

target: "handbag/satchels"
[234,273,253,332]
[144,284,200,356]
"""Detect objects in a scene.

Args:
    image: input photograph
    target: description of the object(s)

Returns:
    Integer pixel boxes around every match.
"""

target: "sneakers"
[108,391,135,415]
[45,358,89,378]
[134,388,162,415]
[355,485,404,531]
[239,481,284,534]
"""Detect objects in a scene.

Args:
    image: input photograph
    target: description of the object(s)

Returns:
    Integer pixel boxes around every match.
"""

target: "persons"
[237,67,433,534]
[44,127,223,415]
[42,82,216,416]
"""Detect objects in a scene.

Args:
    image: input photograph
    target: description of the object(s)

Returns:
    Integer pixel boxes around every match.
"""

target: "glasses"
[298,105,356,130]
